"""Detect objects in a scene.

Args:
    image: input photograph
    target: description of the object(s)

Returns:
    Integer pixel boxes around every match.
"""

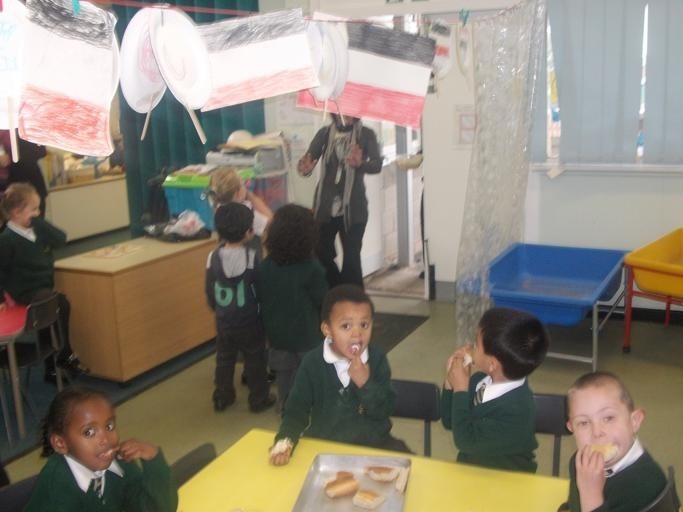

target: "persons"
[555,373,683,512]
[205,167,275,383]
[205,203,277,413]
[394,147,424,280]
[20,387,178,512]
[271,283,416,468]
[442,306,548,471]
[297,115,382,289]
[1,129,52,219]
[2,184,90,386]
[258,204,327,416]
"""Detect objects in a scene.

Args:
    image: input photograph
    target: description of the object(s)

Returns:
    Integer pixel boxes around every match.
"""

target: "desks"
[53,230,217,389]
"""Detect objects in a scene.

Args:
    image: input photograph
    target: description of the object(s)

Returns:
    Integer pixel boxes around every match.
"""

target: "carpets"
[362,313,431,354]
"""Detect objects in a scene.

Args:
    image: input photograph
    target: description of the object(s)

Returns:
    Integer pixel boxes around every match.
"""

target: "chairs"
[640,466,681,511]
[0,291,63,418]
[1,476,41,510]
[172,443,217,489]
[392,378,441,458]
[532,392,573,476]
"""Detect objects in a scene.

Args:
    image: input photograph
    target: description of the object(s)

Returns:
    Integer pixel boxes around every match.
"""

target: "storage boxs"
[254,173,289,212]
[161,167,255,230]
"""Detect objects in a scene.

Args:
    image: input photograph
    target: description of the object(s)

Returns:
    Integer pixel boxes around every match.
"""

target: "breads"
[354,492,386,509]
[589,442,619,464]
[367,465,400,480]
[324,472,359,498]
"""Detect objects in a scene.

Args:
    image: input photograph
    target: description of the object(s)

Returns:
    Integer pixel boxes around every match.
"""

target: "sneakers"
[248,393,277,413]
[55,353,89,377]
[213,392,236,412]
[42,367,75,387]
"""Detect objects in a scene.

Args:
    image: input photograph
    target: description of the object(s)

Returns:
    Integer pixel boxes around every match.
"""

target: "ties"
[88,477,105,507]
[473,382,487,407]
